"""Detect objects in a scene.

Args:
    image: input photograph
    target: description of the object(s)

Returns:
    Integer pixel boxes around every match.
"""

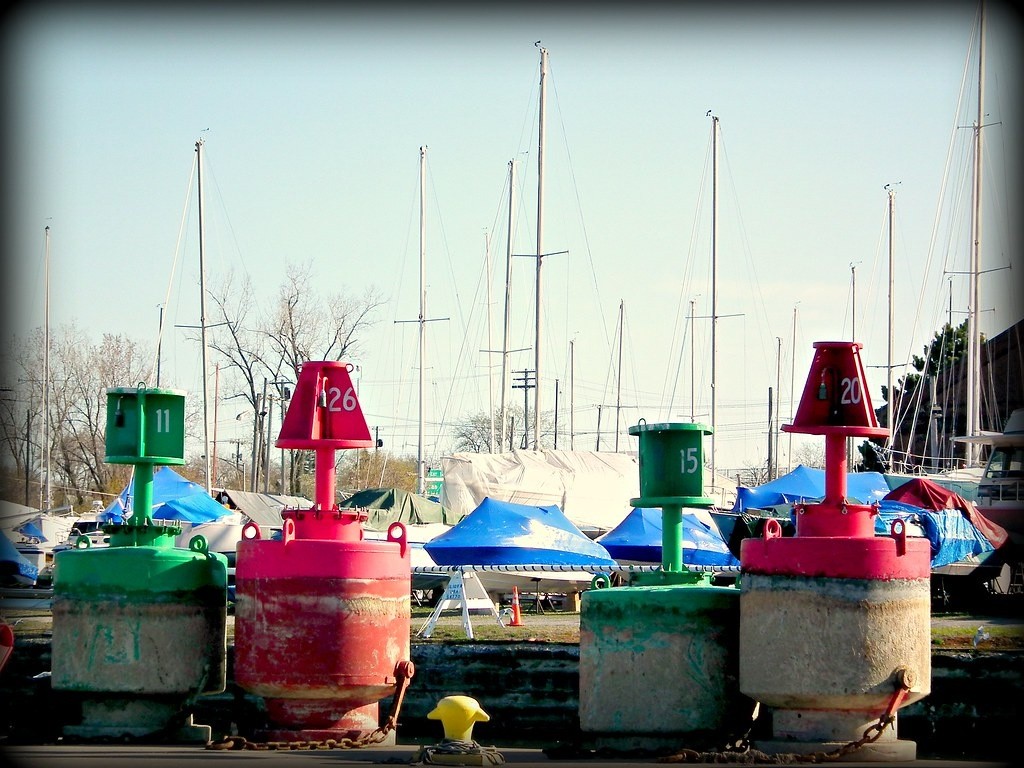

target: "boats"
[421,496,619,595]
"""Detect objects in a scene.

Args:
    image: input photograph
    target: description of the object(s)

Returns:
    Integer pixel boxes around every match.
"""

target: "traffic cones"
[506,586,525,628]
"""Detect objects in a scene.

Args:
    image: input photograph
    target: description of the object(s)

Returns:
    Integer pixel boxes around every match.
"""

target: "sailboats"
[0,0,1024,591]
